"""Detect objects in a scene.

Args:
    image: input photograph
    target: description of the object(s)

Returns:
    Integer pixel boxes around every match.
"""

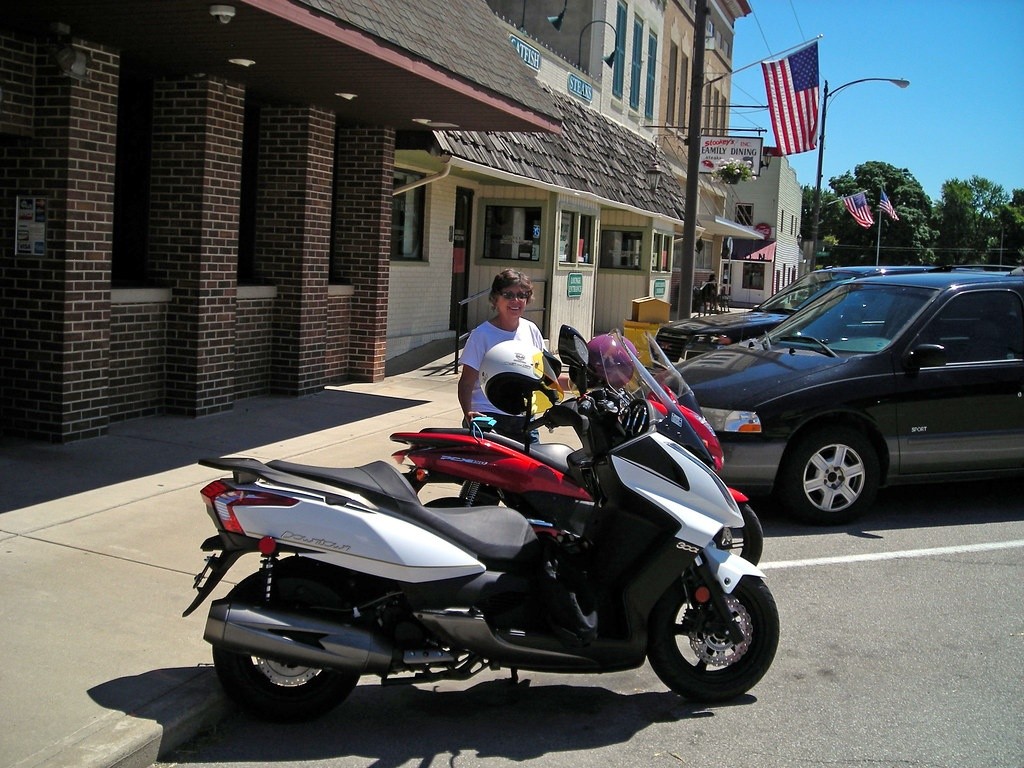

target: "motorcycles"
[379,334,765,567]
[180,324,780,728]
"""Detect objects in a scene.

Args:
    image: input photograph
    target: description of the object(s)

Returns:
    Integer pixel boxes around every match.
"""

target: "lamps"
[522,0,567,42]
[577,20,619,72]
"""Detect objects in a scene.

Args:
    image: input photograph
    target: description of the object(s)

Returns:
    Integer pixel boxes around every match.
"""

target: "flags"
[880,188,898,221]
[762,37,821,155]
[842,192,876,229]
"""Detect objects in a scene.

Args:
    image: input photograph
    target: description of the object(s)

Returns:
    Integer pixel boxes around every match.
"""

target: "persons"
[457,267,576,506]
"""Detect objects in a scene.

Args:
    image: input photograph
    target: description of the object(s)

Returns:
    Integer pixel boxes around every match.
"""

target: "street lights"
[810,77,911,270]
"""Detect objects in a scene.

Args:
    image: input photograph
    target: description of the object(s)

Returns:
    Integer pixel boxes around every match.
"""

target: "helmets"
[587,334,637,387]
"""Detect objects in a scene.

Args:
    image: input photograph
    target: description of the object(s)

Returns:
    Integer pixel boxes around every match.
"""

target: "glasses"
[499,290,528,300]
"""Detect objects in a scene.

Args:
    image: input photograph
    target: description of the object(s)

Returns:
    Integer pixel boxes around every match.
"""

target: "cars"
[649,262,944,370]
[643,271,1024,526]
